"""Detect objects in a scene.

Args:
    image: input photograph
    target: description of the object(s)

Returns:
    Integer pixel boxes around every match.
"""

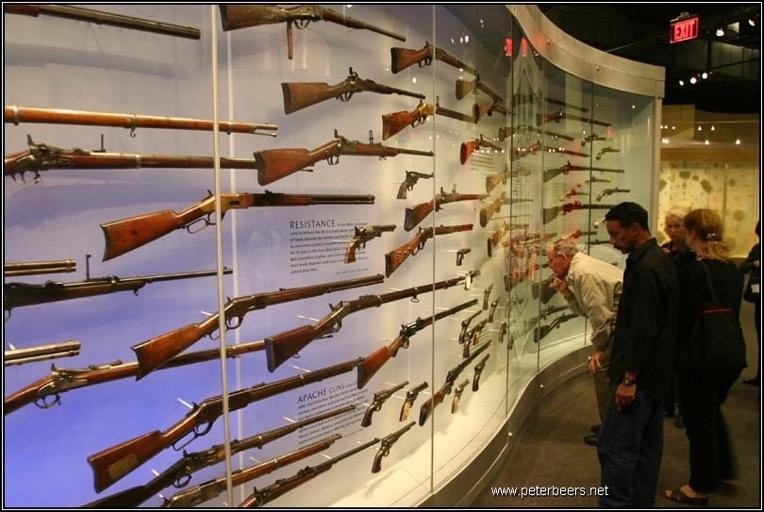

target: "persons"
[547,202,761,508]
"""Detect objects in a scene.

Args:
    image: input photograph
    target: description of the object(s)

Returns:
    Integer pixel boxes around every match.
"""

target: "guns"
[3,4,631,509]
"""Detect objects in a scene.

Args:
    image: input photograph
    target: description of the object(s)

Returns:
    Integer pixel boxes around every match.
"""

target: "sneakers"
[743,376,761,387]
[663,403,687,429]
[583,423,604,446]
[712,480,742,498]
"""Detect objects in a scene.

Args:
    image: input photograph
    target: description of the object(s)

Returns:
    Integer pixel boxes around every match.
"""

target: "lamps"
[678,53,761,86]
[705,11,760,40]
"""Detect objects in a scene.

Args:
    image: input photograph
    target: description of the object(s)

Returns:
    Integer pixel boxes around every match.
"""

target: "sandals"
[661,485,709,506]
